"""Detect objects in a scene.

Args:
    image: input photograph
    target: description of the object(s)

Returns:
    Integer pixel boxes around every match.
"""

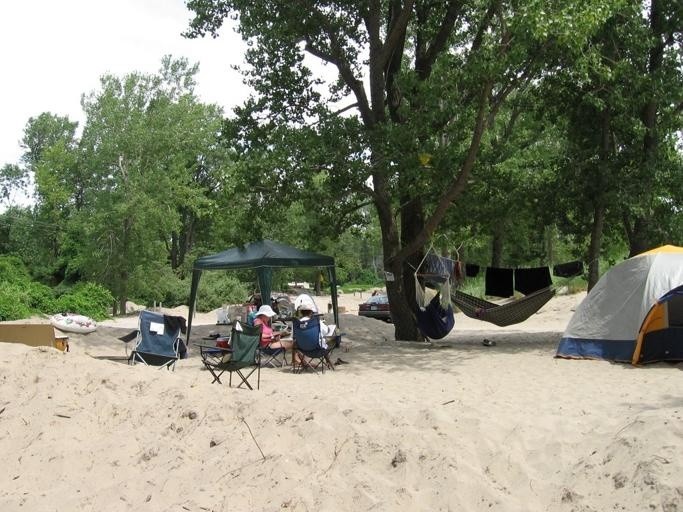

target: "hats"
[255,304,277,317]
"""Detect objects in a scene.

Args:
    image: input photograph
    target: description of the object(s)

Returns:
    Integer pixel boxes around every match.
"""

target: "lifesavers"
[50,312,96,333]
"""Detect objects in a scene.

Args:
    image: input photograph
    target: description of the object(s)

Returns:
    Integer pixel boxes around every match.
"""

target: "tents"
[554,245,683,365]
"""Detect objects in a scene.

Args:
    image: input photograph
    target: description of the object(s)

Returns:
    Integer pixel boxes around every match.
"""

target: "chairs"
[194,308,287,391]
[117,309,186,372]
[277,313,345,373]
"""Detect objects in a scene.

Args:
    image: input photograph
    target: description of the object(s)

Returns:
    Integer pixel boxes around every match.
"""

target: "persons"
[253,305,314,367]
[298,303,335,370]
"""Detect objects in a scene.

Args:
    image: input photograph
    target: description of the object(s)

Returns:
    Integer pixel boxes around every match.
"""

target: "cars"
[357,291,391,323]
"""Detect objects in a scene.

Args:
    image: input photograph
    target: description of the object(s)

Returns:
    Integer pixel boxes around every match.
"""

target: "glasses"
[298,302,314,311]
